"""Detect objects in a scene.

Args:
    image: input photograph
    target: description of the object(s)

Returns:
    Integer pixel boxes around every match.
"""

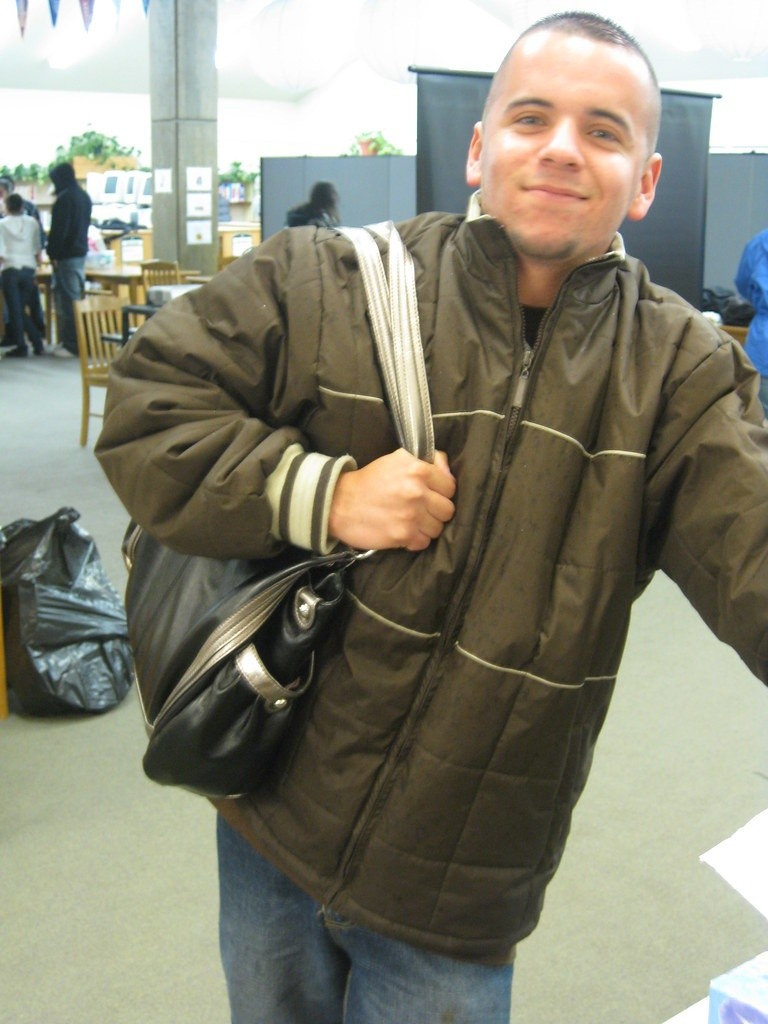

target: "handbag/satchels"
[0,507,134,718]
[702,287,756,326]
[120,518,357,800]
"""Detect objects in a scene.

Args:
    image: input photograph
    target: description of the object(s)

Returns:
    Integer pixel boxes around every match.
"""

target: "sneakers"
[34,348,42,354]
[52,347,74,357]
[6,349,27,357]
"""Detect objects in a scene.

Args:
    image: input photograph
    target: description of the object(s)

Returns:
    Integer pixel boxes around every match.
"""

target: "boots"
[0,324,17,345]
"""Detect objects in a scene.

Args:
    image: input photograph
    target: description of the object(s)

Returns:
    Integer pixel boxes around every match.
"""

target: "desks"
[34,265,213,348]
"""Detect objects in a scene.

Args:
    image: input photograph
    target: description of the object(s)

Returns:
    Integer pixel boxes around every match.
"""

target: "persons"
[1,164,94,357]
[735,229,768,421]
[286,180,340,226]
[94,11,768,1023]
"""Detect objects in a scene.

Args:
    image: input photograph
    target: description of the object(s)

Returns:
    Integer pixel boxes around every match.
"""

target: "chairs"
[142,259,180,322]
[74,296,123,447]
